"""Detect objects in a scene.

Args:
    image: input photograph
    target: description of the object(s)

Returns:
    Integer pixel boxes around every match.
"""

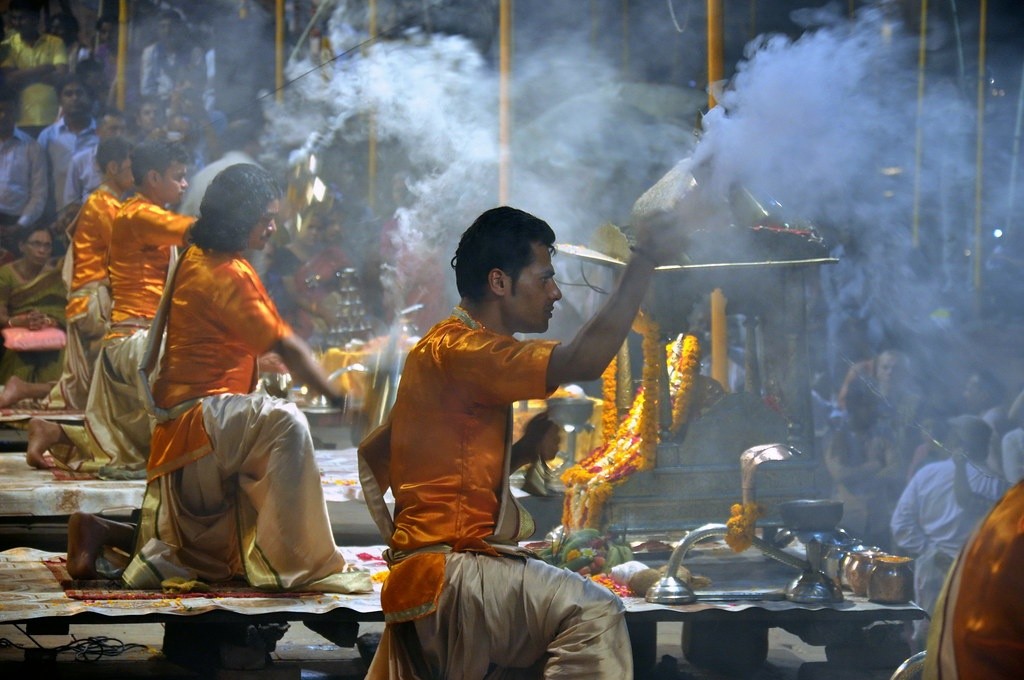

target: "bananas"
[538,527,633,576]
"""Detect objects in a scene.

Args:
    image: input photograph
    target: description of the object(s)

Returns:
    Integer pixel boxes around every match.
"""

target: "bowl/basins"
[777,499,847,533]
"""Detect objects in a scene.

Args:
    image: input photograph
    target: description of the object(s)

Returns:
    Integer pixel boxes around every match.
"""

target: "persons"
[37,77,97,232]
[358,206,694,680]
[1,134,136,409]
[695,319,1024,552]
[177,118,272,286]
[888,415,1011,649]
[1,225,66,385]
[0,0,321,119]
[127,97,179,185]
[0,86,49,259]
[65,164,375,595]
[138,11,208,109]
[821,220,891,393]
[0,0,67,139]
[27,137,200,479]
[169,88,227,171]
[64,104,136,208]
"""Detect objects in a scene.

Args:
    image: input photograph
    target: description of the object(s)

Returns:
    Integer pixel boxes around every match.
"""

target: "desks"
[0,383,926,674]
